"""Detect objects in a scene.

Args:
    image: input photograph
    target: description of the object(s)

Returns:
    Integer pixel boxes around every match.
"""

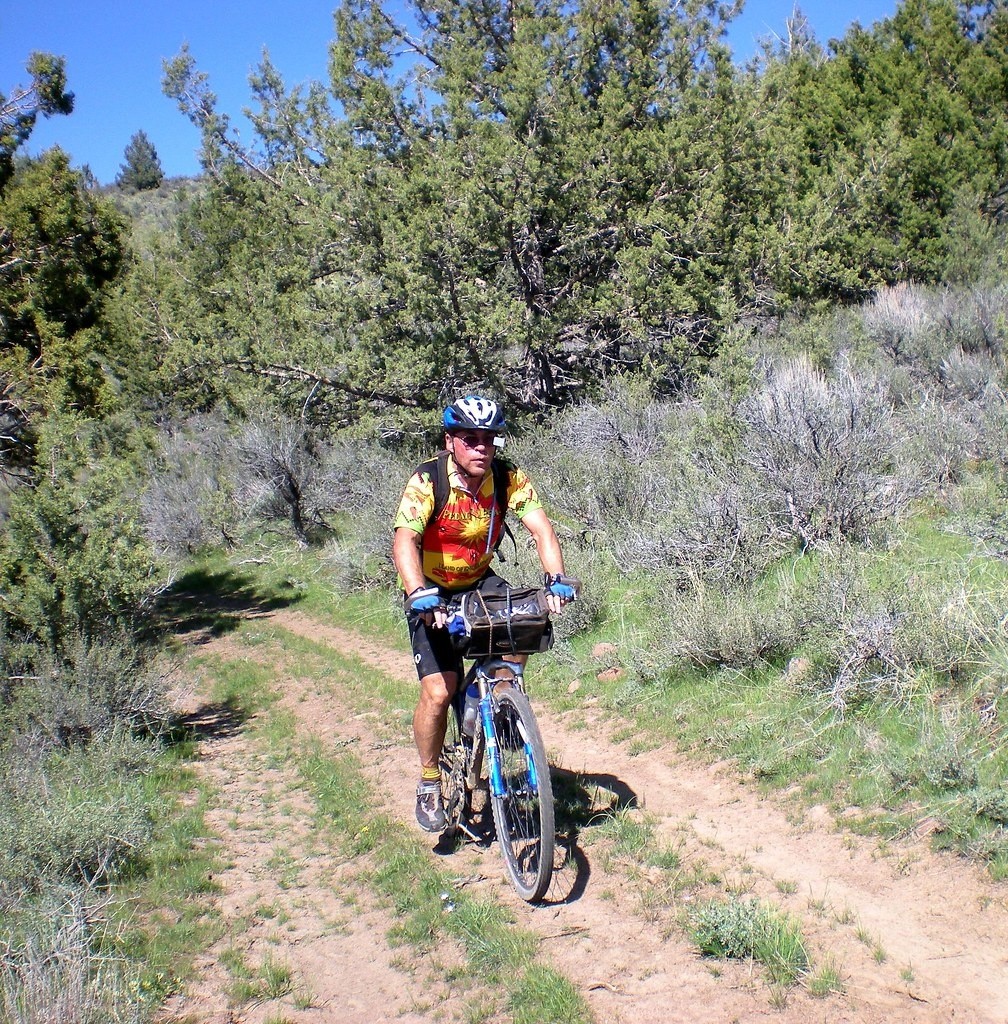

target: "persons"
[393,394,575,833]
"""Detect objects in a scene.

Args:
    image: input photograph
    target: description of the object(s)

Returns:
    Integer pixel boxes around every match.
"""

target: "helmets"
[443,395,506,432]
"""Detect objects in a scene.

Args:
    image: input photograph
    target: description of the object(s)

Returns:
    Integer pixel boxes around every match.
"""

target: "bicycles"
[409,599,555,902]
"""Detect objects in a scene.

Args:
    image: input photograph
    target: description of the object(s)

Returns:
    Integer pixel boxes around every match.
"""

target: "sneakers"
[493,705,524,751]
[416,778,446,832]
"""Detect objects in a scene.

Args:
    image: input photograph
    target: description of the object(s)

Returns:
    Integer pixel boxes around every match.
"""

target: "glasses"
[450,433,497,447]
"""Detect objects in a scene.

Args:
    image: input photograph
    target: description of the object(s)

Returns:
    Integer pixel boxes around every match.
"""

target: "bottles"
[463,682,479,738]
